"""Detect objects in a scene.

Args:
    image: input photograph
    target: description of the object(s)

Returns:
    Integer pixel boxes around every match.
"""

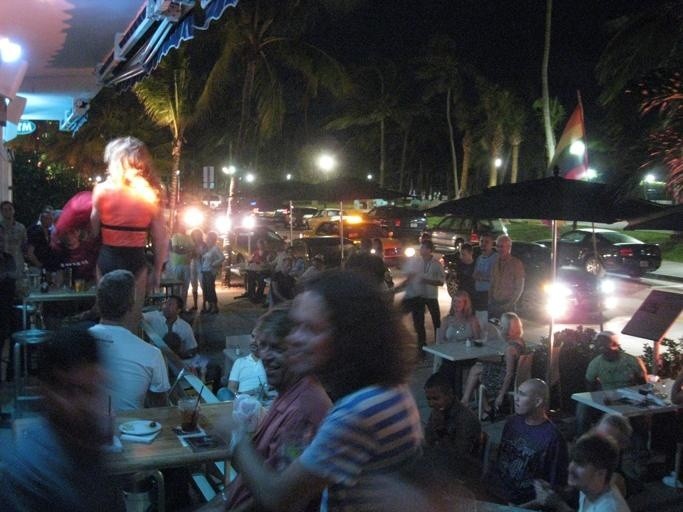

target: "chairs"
[547,343,563,408]
[432,321,500,373]
[478,354,533,423]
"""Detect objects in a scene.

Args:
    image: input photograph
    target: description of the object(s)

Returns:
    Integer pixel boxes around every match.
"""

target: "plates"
[119,420,162,435]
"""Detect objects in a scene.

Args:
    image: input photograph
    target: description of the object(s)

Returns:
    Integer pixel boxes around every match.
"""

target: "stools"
[12,329,53,407]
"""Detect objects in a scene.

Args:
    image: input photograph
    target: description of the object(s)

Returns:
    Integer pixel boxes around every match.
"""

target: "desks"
[422,338,539,401]
[22,279,272,488]
[571,378,681,451]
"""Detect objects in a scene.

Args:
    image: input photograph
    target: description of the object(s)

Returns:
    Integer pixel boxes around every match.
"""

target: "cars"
[368,204,428,240]
[531,228,661,278]
[418,214,512,255]
[256,205,365,230]
[442,239,616,323]
[303,219,415,269]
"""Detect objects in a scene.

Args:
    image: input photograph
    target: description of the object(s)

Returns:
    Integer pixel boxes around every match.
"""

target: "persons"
[1,135,683,512]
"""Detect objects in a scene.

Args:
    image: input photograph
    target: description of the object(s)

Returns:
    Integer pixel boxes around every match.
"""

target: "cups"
[75,279,85,292]
[178,396,201,433]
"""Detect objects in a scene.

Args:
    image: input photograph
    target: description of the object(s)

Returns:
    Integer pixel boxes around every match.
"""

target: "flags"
[541,105,589,223]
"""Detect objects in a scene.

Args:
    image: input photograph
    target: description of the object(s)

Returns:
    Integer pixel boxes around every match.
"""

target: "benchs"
[22,279,272,488]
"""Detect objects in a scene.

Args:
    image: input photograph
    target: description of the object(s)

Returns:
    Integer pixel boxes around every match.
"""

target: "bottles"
[40,266,49,293]
[235,343,240,356]
[466,337,471,348]
